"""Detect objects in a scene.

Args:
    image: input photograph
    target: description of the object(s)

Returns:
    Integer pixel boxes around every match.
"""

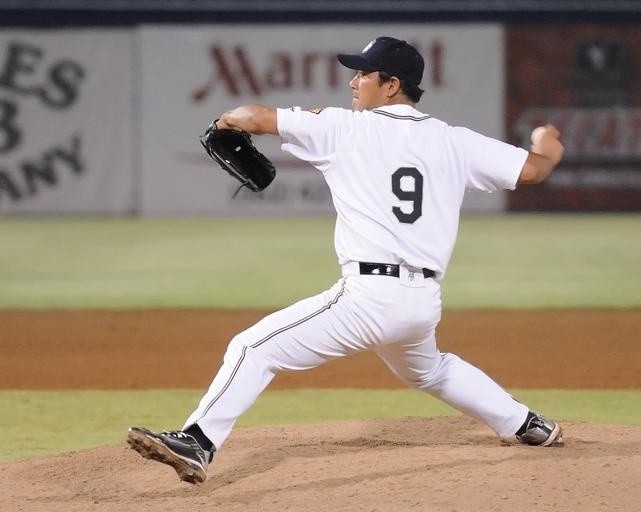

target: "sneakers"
[126,427,213,482]
[514,411,559,446]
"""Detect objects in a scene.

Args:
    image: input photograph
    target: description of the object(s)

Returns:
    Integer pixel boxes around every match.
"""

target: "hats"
[336,36,423,84]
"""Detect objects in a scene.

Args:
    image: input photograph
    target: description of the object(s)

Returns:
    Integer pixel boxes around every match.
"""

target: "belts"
[358,262,435,278]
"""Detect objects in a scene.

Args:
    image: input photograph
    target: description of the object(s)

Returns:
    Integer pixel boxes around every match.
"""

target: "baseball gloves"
[199,119,276,193]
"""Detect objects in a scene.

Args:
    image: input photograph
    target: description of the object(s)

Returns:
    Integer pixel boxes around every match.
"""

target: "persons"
[127,36,564,484]
